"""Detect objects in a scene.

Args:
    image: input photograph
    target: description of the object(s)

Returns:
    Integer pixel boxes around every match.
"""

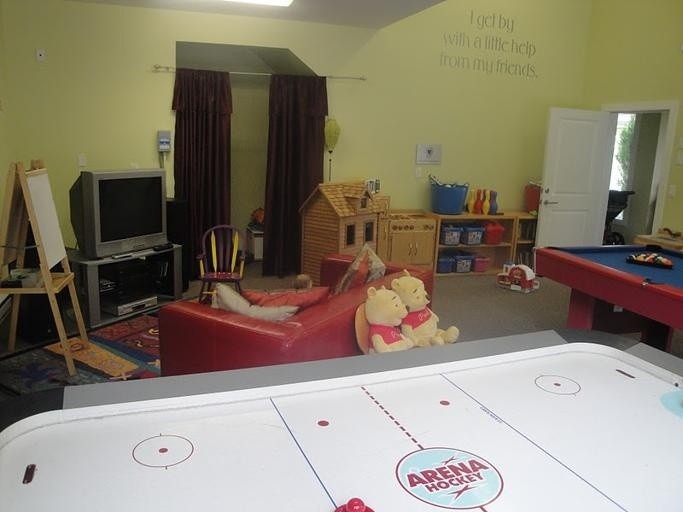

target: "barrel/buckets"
[431,183,469,213]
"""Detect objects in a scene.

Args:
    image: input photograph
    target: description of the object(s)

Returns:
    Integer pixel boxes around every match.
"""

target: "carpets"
[0,315,162,395]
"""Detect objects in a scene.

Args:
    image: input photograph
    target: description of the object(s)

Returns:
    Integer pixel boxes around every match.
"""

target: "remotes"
[152,243,173,252]
[112,252,131,259]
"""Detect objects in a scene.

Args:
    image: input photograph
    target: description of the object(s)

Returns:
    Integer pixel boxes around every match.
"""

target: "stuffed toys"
[366,269,459,355]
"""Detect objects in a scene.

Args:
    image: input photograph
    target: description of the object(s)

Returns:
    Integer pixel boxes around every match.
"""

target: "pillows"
[210,241,388,324]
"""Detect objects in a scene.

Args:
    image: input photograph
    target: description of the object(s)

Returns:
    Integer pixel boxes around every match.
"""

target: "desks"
[533,243,682,352]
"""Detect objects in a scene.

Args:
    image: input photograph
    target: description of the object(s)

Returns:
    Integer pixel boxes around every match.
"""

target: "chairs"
[196,225,248,306]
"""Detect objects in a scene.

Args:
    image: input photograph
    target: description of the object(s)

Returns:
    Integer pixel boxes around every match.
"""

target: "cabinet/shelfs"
[429,211,537,277]
[391,232,432,266]
[65,241,183,331]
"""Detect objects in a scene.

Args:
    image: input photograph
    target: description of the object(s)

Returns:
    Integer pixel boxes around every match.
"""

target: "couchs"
[153,251,434,376]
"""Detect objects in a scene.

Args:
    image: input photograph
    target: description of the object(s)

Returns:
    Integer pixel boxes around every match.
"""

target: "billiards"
[627,251,672,265]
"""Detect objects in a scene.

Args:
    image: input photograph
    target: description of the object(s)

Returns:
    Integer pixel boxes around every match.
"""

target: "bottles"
[467,188,497,215]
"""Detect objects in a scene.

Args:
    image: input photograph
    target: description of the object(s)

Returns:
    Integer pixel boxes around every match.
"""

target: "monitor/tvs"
[69,168,168,258]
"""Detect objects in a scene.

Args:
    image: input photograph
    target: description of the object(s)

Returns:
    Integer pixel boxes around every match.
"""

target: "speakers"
[7,221,64,342]
[158,198,190,295]
